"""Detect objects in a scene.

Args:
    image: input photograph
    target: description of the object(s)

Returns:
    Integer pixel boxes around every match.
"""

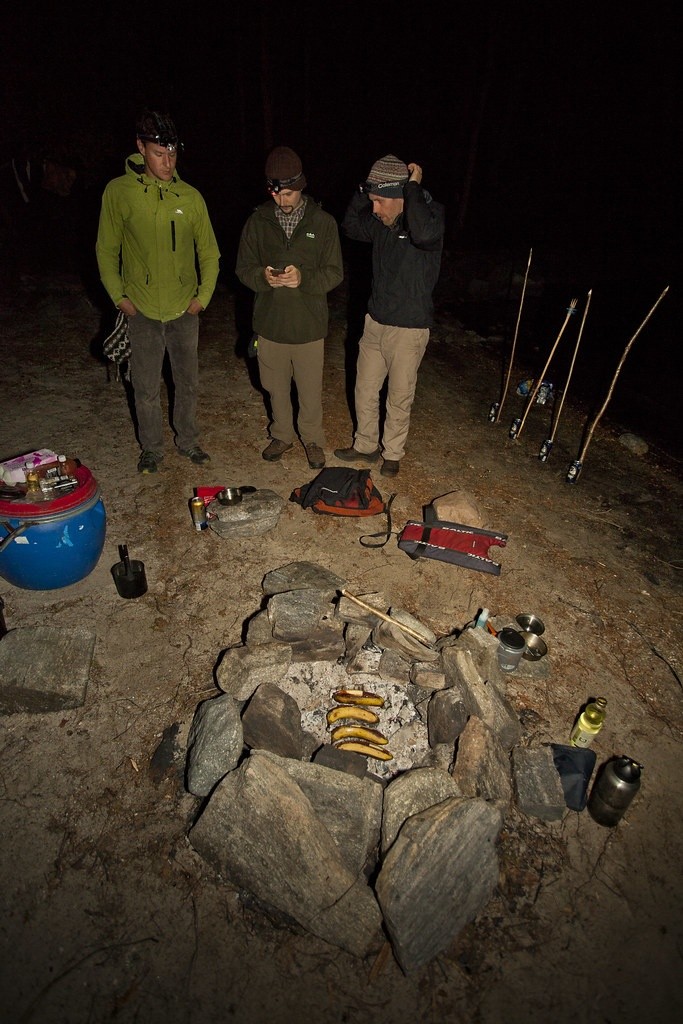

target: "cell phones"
[271,269,285,277]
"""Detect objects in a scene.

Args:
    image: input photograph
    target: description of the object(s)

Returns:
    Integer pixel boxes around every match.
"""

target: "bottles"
[570,696,608,749]
[475,608,490,628]
[587,754,643,828]
[56,454,74,481]
[496,627,526,673]
[24,461,40,492]
[35,458,81,480]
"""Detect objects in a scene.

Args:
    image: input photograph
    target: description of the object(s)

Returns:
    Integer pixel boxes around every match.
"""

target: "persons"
[95,115,222,475]
[335,154,446,479]
[235,147,344,469]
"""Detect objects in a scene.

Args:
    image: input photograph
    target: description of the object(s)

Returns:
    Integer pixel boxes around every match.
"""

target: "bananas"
[326,706,380,730]
[334,741,393,760]
[331,725,389,744]
[332,690,384,706]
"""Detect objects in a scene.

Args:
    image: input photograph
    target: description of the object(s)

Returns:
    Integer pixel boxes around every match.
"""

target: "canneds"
[488,402,500,422]
[509,418,522,439]
[566,459,581,484]
[538,439,553,461]
[192,497,208,530]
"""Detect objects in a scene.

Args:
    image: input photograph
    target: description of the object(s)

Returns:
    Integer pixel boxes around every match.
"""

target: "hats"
[102,310,132,383]
[266,147,306,192]
[365,154,409,198]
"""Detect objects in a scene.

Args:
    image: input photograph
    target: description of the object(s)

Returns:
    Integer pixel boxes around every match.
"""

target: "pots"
[110,545,148,599]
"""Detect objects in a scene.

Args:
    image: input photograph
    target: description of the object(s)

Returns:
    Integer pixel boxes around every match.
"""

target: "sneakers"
[305,442,326,469]
[334,446,380,463]
[380,460,399,477]
[137,449,164,473]
[262,439,294,462]
[177,446,211,464]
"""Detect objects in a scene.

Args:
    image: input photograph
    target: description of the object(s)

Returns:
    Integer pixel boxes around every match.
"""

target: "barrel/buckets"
[0,485,107,591]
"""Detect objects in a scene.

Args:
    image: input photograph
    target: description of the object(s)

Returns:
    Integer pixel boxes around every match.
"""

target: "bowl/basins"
[517,631,547,662]
[515,613,546,636]
[216,488,242,506]
